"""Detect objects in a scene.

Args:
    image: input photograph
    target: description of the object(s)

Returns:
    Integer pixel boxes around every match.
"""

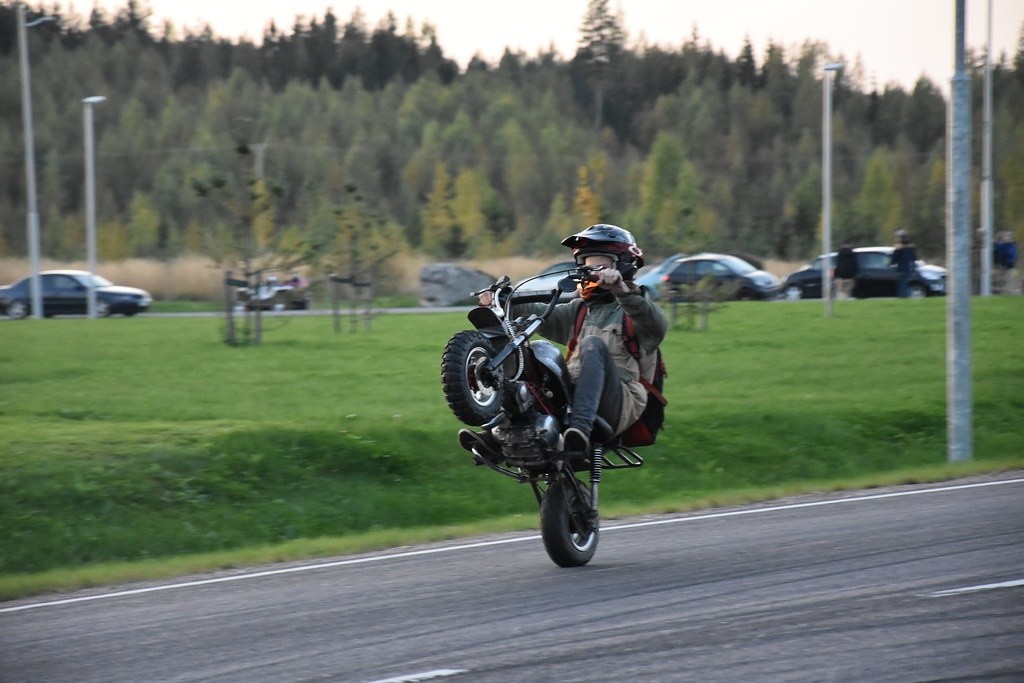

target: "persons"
[830,239,858,300]
[994,228,1006,294]
[888,230,921,298]
[998,229,1017,295]
[457,223,670,466]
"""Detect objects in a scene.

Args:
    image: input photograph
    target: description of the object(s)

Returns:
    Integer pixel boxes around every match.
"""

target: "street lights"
[82,96,108,317]
[820,61,844,301]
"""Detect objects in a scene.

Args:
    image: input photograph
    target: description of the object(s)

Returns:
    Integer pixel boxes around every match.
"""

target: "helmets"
[561,224,644,302]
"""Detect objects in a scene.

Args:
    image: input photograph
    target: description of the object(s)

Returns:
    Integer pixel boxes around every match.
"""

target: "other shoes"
[563,428,592,452]
[459,429,505,464]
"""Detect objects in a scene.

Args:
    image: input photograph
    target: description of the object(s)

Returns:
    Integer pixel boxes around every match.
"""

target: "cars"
[786,246,946,301]
[0,269,153,318]
[512,260,669,301]
[660,254,784,303]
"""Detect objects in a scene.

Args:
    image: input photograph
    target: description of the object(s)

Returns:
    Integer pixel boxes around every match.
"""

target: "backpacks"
[569,285,667,446]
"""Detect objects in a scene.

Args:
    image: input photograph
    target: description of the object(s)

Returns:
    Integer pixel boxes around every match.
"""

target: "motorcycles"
[439,263,644,568]
